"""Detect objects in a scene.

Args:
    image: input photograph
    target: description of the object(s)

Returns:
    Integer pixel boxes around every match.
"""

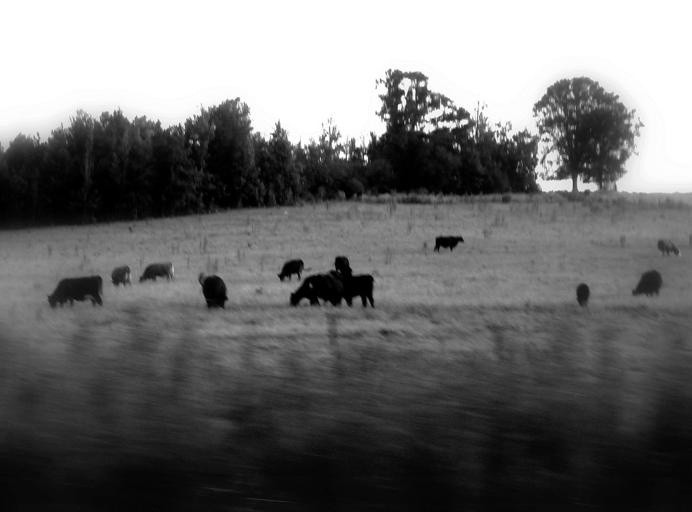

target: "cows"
[576,283,589,307]
[658,239,680,256]
[111,265,132,287]
[47,275,103,308]
[277,256,376,309]
[198,272,228,310]
[139,262,175,282]
[434,236,464,253]
[632,270,662,297]
